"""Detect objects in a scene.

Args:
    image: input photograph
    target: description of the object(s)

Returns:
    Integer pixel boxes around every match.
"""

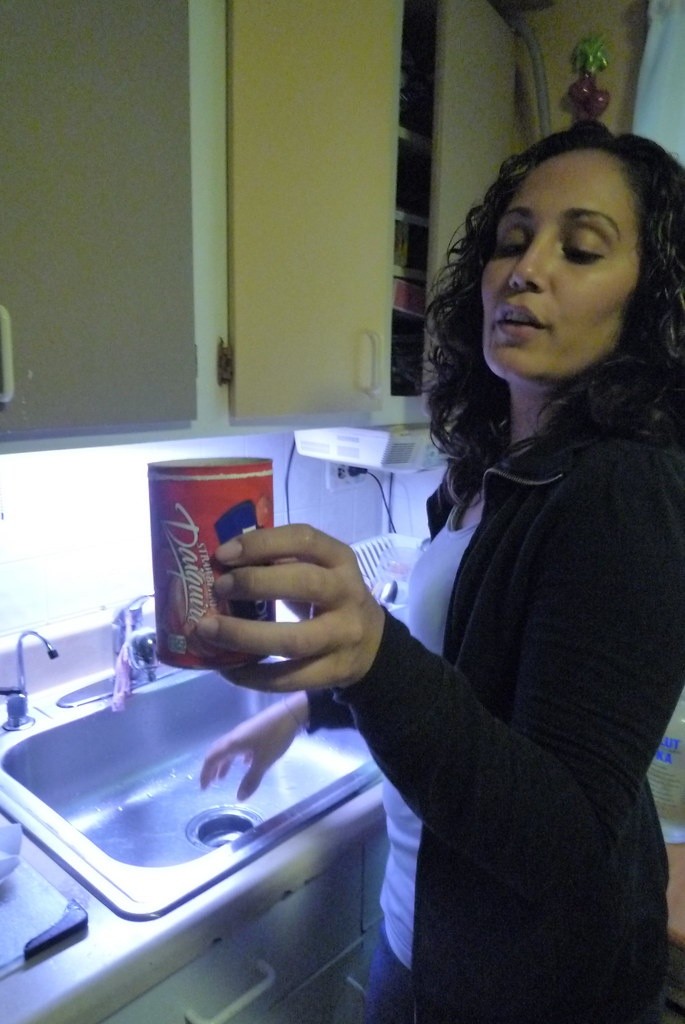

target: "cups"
[147,456,274,671]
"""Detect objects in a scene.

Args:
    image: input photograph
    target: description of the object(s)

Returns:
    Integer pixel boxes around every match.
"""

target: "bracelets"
[282,698,300,727]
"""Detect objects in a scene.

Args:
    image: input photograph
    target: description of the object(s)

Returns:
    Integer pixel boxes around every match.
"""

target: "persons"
[196,122,685,1024]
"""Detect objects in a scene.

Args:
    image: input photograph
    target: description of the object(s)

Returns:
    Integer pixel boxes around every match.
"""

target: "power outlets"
[325,462,368,492]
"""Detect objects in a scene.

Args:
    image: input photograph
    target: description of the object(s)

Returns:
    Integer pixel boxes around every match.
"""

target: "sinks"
[0,656,383,922]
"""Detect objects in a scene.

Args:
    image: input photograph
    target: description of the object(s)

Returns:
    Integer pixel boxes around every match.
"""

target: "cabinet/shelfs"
[98,830,388,1024]
[0,0,198,444]
[226,0,648,420]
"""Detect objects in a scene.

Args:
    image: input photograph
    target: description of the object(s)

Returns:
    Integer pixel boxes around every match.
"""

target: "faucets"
[2,629,58,731]
[114,595,155,682]
